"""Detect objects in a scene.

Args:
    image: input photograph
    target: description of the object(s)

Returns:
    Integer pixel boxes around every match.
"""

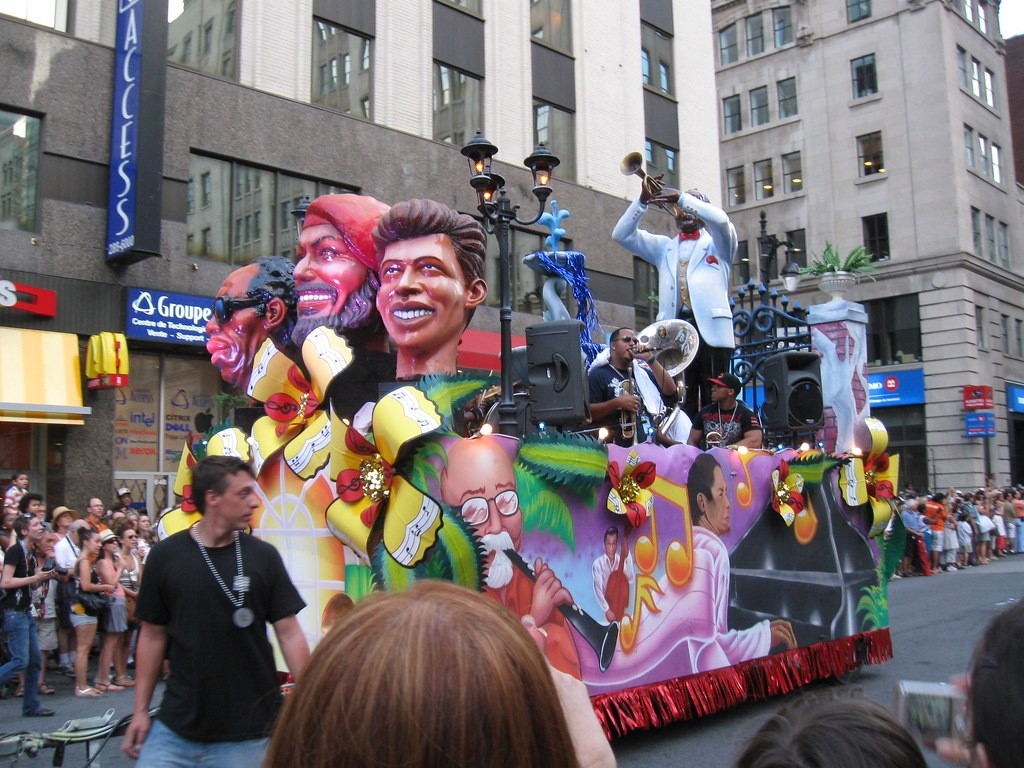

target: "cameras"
[41,557,68,576]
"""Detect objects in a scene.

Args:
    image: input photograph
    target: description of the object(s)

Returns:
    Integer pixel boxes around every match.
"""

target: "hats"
[118,488,131,498]
[706,372,741,394]
[52,506,80,530]
[100,529,118,542]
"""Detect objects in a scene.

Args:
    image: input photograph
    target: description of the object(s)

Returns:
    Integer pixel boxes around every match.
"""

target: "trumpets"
[618,368,638,445]
[700,411,723,451]
[619,151,688,217]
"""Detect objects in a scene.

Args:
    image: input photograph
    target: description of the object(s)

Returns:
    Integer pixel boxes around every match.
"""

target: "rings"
[635,405,637,408]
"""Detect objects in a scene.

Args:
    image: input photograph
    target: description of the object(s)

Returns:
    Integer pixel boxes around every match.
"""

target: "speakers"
[524,318,590,428]
[763,352,825,431]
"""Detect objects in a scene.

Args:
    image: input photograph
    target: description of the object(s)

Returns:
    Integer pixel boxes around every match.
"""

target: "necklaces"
[192,520,254,627]
[718,401,739,439]
[609,364,626,380]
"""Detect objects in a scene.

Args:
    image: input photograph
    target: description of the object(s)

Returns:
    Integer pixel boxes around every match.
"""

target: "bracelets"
[647,355,656,365]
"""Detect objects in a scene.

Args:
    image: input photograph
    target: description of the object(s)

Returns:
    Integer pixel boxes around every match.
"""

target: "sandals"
[14,661,138,717]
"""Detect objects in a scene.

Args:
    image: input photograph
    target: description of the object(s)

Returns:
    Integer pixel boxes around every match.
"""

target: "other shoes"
[887,550,1016,581]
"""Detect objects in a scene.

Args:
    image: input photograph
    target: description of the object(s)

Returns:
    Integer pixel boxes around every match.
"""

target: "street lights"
[459,127,561,440]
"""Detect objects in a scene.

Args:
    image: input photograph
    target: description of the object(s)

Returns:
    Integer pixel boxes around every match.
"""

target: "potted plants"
[798,238,880,303]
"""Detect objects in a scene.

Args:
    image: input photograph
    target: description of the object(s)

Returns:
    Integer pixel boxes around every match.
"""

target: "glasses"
[105,539,119,543]
[613,337,638,345]
[24,513,31,518]
[122,535,138,540]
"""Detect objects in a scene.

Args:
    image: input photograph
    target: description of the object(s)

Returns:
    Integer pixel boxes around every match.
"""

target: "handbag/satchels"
[125,594,142,626]
[78,590,107,616]
[978,514,995,533]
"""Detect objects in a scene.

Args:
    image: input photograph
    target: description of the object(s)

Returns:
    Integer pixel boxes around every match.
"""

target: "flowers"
[771,457,805,527]
[157,326,444,568]
[839,416,899,538]
[607,449,657,531]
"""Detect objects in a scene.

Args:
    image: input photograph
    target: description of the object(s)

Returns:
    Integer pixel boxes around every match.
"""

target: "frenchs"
[633,318,702,436]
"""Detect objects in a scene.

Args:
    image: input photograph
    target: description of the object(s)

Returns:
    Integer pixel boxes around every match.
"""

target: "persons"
[206,254,296,394]
[890,483,1024,580]
[588,326,680,449]
[288,192,392,355]
[611,152,737,417]
[257,577,619,768]
[372,197,487,382]
[686,373,762,455]
[932,599,1024,768]
[0,471,171,717]
[735,690,928,768]
[122,455,313,768]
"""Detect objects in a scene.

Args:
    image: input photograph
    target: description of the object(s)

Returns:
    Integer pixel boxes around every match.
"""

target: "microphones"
[632,347,662,354]
